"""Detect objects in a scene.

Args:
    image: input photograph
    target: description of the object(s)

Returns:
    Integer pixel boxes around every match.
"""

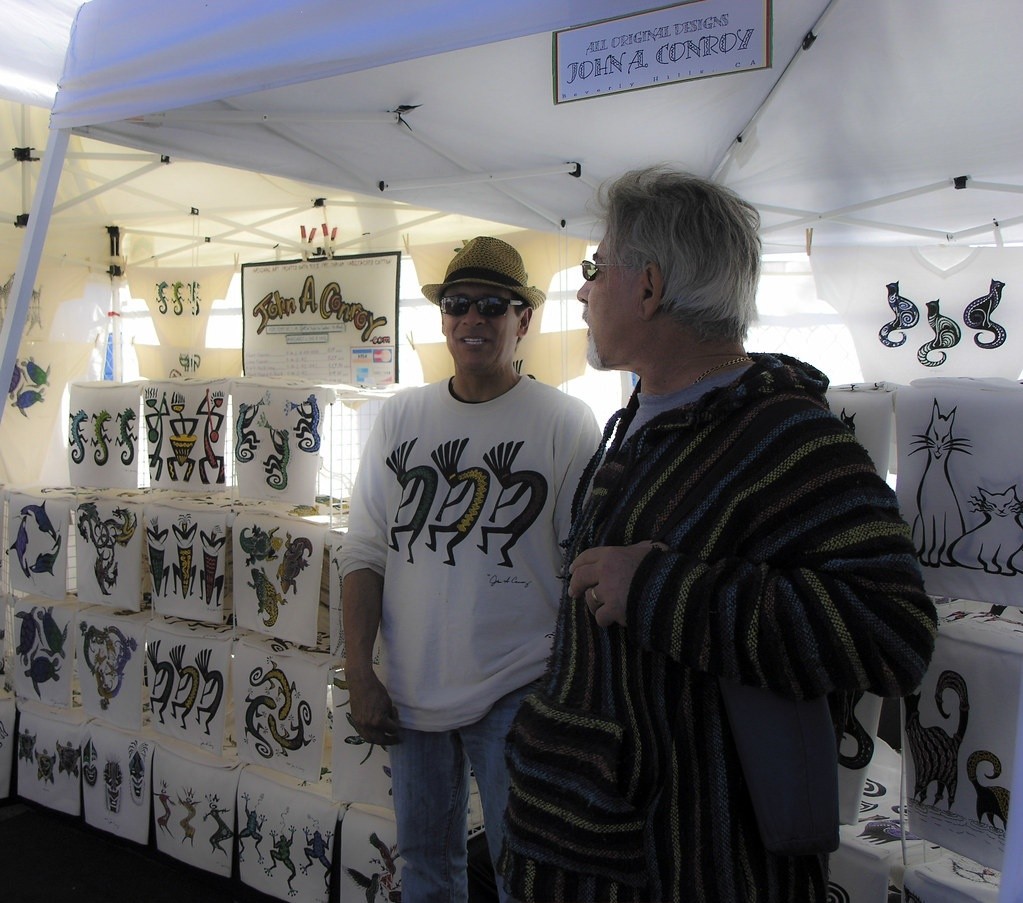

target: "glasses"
[577,260,636,283]
[439,295,522,316]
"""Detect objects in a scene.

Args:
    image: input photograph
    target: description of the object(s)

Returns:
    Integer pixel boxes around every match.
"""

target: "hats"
[421,236,548,311]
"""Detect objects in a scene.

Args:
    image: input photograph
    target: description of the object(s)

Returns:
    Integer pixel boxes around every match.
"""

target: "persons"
[496,163,938,903]
[336,233,602,902]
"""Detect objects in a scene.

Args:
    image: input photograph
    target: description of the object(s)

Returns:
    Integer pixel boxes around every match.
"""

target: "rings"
[590,588,600,604]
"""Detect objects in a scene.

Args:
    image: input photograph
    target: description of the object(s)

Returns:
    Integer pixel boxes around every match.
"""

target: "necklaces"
[691,355,754,386]
